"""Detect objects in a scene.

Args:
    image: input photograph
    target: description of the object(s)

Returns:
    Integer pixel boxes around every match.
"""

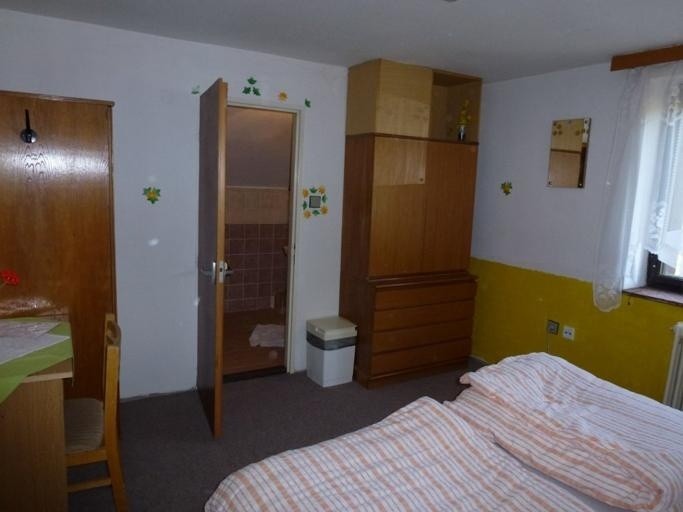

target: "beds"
[228,353,682,512]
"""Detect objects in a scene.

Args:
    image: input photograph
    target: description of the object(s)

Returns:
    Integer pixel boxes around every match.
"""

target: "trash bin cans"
[305,316,358,387]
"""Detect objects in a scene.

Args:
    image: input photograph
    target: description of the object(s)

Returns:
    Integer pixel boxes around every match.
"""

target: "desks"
[0,316,74,512]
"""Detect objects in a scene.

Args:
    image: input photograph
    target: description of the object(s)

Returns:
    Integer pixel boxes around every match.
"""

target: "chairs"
[64,313,130,512]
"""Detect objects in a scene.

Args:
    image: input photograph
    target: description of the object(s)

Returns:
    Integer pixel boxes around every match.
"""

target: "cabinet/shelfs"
[2,89,120,400]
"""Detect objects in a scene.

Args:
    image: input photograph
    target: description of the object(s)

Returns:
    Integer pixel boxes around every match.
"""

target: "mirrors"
[546,118,591,189]
